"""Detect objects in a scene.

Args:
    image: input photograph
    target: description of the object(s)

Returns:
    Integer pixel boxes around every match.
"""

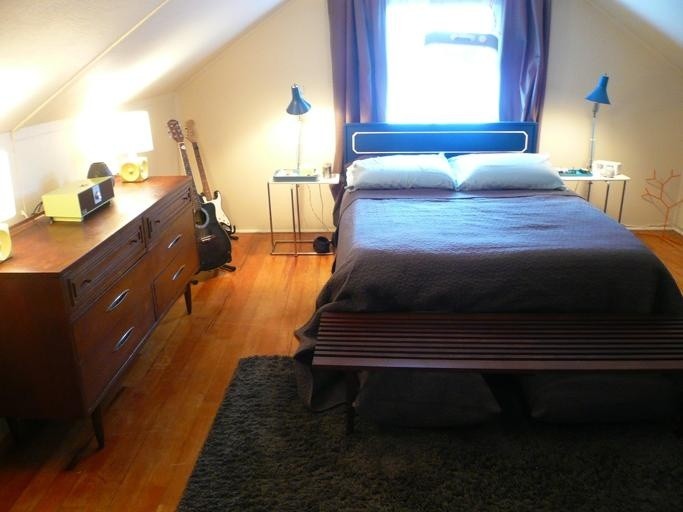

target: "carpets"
[176,356,682,512]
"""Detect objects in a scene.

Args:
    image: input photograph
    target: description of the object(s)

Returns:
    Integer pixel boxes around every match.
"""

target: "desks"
[559,172,631,223]
[0,175,201,450]
[267,173,341,256]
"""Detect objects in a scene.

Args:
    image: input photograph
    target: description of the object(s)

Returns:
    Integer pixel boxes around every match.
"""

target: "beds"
[294,121,683,413]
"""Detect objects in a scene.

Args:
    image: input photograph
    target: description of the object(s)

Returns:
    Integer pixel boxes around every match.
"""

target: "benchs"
[312,313,683,434]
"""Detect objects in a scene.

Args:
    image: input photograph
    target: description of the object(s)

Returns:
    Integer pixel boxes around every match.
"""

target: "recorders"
[41,176,115,222]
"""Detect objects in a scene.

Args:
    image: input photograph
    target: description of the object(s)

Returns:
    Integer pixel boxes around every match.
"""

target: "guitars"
[166,118,232,271]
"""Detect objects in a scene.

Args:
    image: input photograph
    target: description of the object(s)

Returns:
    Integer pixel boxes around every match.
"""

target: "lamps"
[584,73,611,168]
[117,110,155,183]
[286,84,312,171]
[0,153,17,263]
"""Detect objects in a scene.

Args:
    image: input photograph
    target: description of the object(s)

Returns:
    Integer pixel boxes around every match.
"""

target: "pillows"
[448,153,568,192]
[352,371,502,429]
[521,373,683,424]
[344,153,456,193]
